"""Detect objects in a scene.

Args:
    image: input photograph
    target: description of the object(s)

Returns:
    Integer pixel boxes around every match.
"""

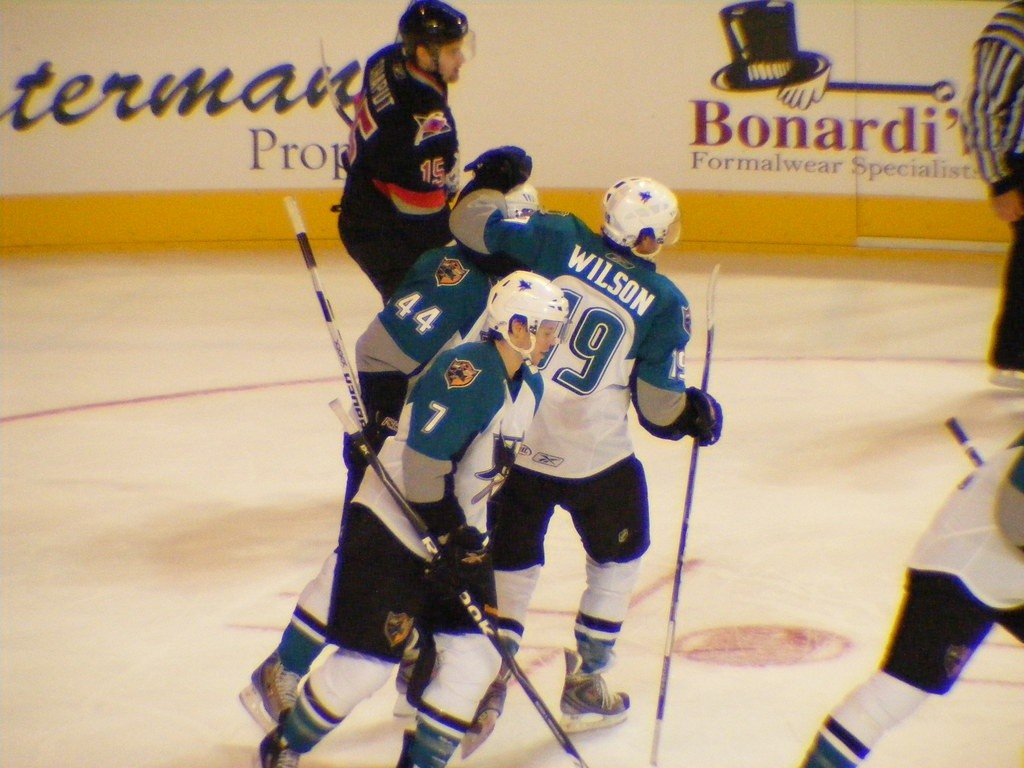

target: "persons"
[448,143,723,735]
[802,432,1024,767]
[337,0,469,310]
[958,0,1024,389]
[253,270,570,768]
[237,181,537,739]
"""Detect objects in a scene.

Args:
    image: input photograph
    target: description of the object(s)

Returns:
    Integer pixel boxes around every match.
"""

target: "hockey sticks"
[318,35,355,131]
[944,414,984,466]
[650,261,723,768]
[327,396,592,767]
[280,192,369,428]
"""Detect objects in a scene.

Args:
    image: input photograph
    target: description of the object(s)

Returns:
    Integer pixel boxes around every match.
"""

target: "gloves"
[463,145,533,195]
[424,524,504,603]
[676,386,723,446]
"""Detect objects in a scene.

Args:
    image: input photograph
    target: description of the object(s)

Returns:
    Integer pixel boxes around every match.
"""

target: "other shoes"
[984,360,1024,387]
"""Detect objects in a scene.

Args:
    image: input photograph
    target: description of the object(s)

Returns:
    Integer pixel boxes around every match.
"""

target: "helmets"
[480,270,569,337]
[398,0,469,43]
[599,176,682,260]
[500,182,539,224]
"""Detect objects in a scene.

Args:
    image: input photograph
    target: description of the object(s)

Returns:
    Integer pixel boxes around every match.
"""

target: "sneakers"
[237,647,312,735]
[392,628,447,718]
[558,647,633,733]
[259,707,301,768]
[460,679,508,760]
